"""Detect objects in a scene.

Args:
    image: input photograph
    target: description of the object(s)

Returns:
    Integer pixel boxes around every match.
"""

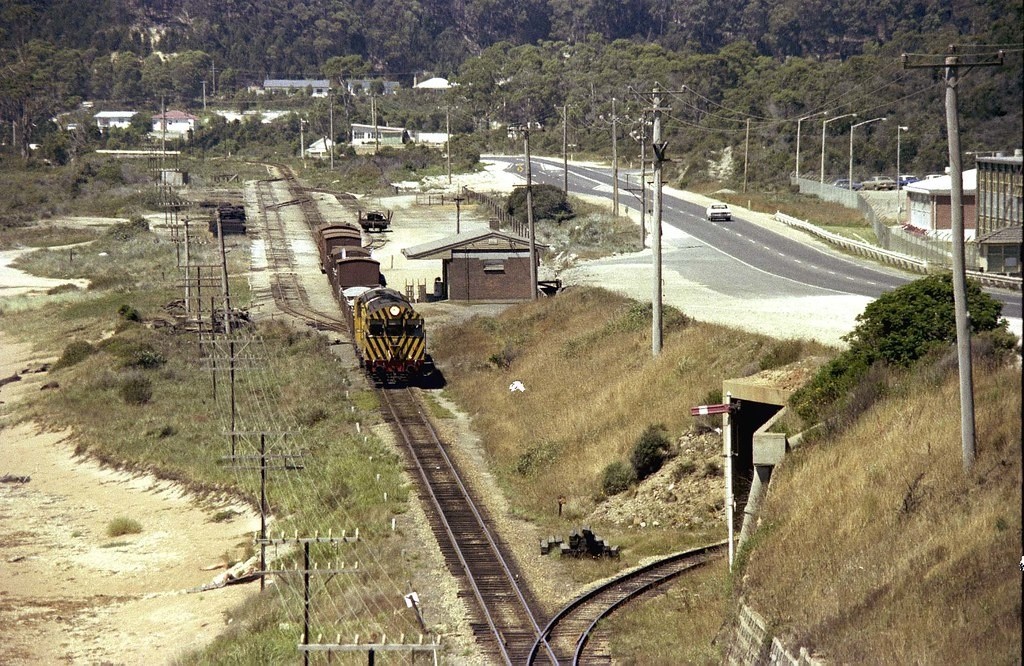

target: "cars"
[707,203,732,223]
[894,175,919,188]
[830,179,861,192]
[922,175,942,181]
[858,176,896,191]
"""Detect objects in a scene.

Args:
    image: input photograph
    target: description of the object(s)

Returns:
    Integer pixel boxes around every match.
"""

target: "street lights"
[795,111,829,186]
[602,98,630,219]
[896,124,908,226]
[622,112,653,248]
[820,113,858,194]
[849,117,888,219]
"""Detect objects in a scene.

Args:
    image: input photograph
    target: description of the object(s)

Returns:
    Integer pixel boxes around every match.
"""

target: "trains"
[316,220,434,388]
